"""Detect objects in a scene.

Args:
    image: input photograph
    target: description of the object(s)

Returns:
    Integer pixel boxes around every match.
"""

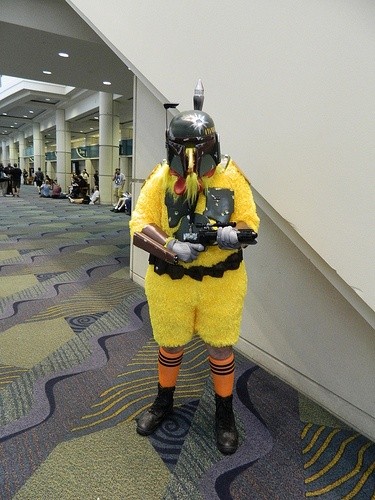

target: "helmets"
[165,110,221,178]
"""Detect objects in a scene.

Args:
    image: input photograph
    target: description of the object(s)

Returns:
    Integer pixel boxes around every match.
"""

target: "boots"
[214,393,239,455]
[137,382,175,437]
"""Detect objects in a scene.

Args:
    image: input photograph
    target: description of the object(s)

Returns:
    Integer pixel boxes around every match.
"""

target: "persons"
[0,163,22,198]
[112,167,126,207]
[128,108,261,456]
[23,168,28,185]
[94,170,99,186]
[45,175,51,182]
[109,192,132,212]
[39,180,51,198]
[68,186,99,204]
[33,167,45,194]
[68,169,89,198]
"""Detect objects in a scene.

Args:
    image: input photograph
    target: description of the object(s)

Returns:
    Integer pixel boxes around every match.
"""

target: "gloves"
[171,241,205,262]
[217,226,241,249]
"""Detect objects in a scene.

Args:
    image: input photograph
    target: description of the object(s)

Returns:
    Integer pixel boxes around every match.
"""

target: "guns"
[173,228,258,252]
